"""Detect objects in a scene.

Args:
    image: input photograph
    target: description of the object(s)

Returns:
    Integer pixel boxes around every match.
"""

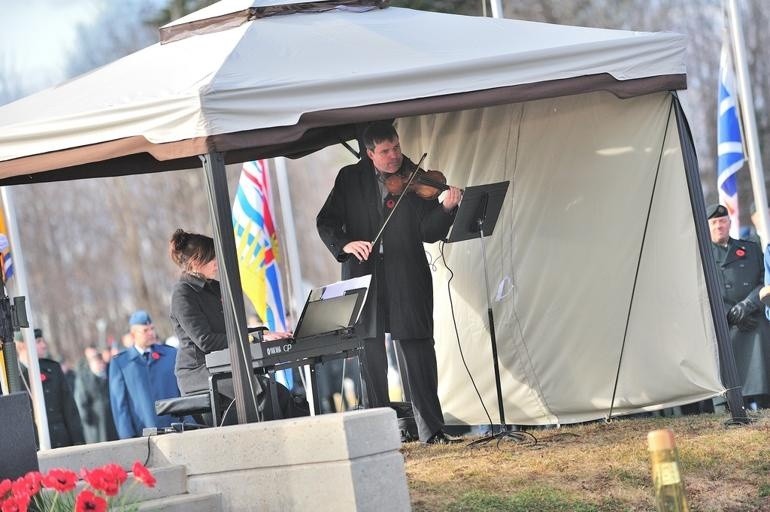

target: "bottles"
[646,428,692,511]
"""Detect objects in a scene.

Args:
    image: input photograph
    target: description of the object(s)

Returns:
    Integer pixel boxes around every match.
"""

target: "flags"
[717,30,749,242]
[0,200,13,282]
[230,156,297,394]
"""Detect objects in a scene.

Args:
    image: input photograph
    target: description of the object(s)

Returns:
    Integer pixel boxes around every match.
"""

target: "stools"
[155,392,235,427]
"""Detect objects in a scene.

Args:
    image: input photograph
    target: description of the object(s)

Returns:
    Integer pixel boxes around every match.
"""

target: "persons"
[315,124,463,446]
[11,310,202,448]
[169,230,294,422]
[706,202,769,412]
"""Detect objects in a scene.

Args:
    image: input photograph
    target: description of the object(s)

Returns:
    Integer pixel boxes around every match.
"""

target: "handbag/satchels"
[351,402,418,443]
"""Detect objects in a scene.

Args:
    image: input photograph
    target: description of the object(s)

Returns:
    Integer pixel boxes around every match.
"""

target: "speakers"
[0,391,40,483]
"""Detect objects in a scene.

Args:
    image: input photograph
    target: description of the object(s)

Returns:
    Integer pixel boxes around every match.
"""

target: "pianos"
[205,326,359,367]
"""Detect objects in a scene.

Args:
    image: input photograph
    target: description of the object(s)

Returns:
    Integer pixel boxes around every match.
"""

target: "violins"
[385,171,464,201]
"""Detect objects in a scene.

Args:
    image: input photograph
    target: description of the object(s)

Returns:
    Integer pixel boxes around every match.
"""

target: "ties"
[143,352,150,361]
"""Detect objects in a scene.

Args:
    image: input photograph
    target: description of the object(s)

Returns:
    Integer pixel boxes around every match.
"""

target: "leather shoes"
[428,430,462,445]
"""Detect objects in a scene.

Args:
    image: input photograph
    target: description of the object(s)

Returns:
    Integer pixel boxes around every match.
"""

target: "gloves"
[728,298,758,331]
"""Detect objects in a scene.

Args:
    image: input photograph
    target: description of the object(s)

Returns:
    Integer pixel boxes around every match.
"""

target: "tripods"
[466,219,526,446]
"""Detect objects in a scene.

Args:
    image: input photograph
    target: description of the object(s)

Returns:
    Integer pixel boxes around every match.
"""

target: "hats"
[130,312,152,324]
[707,205,728,220]
